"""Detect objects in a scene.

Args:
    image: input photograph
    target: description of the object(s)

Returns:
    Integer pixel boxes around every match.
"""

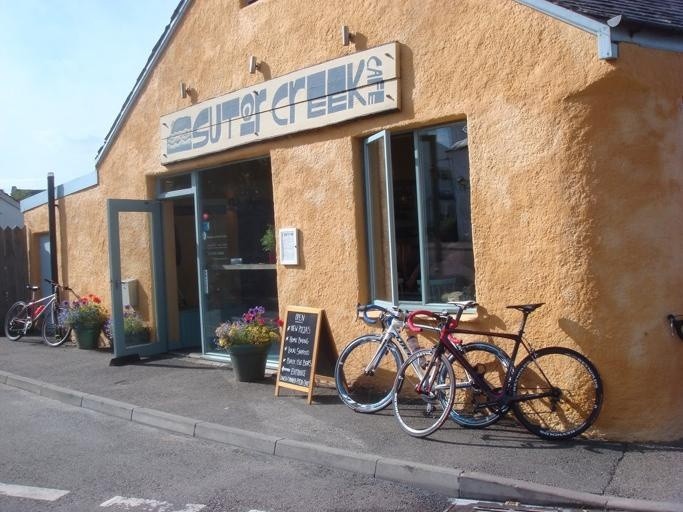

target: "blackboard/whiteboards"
[276,306,324,393]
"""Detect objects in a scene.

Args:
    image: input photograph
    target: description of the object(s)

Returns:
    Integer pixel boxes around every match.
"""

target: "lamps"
[178,81,189,98]
[340,25,355,47]
[248,55,259,74]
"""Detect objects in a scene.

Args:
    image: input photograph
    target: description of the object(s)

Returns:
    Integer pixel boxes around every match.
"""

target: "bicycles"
[5,279,75,345]
[390,301,606,445]
[335,303,517,429]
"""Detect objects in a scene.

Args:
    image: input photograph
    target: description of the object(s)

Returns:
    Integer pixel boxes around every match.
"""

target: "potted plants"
[258,224,275,265]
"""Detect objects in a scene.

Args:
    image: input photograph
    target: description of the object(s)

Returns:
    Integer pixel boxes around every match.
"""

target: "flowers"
[210,303,282,351]
[102,302,149,341]
[56,295,109,330]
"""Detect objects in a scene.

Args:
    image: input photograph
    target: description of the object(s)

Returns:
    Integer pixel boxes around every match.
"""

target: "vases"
[108,334,149,355]
[73,321,101,348]
[224,343,271,382]
